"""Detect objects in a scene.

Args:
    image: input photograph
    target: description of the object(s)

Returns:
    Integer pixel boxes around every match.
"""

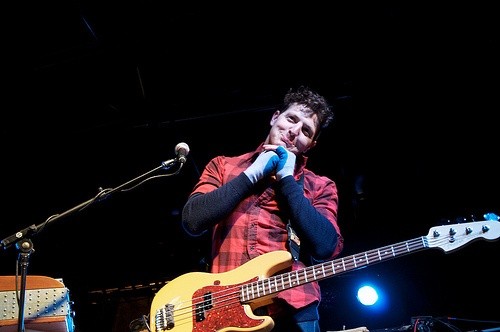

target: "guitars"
[149,214,500,332]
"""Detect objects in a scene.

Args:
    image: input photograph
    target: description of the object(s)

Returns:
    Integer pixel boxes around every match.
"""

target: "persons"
[182,85,344,332]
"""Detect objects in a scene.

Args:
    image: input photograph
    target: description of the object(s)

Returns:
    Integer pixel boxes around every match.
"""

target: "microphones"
[175,142,189,163]
